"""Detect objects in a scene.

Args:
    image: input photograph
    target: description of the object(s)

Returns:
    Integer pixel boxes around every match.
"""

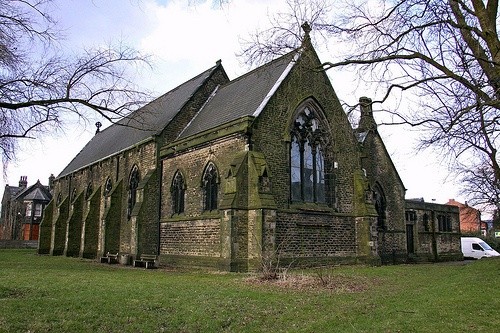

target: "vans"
[460,236,500,259]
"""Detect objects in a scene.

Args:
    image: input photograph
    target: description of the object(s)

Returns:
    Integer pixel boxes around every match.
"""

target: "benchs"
[133,254,157,270]
[100,251,119,264]
